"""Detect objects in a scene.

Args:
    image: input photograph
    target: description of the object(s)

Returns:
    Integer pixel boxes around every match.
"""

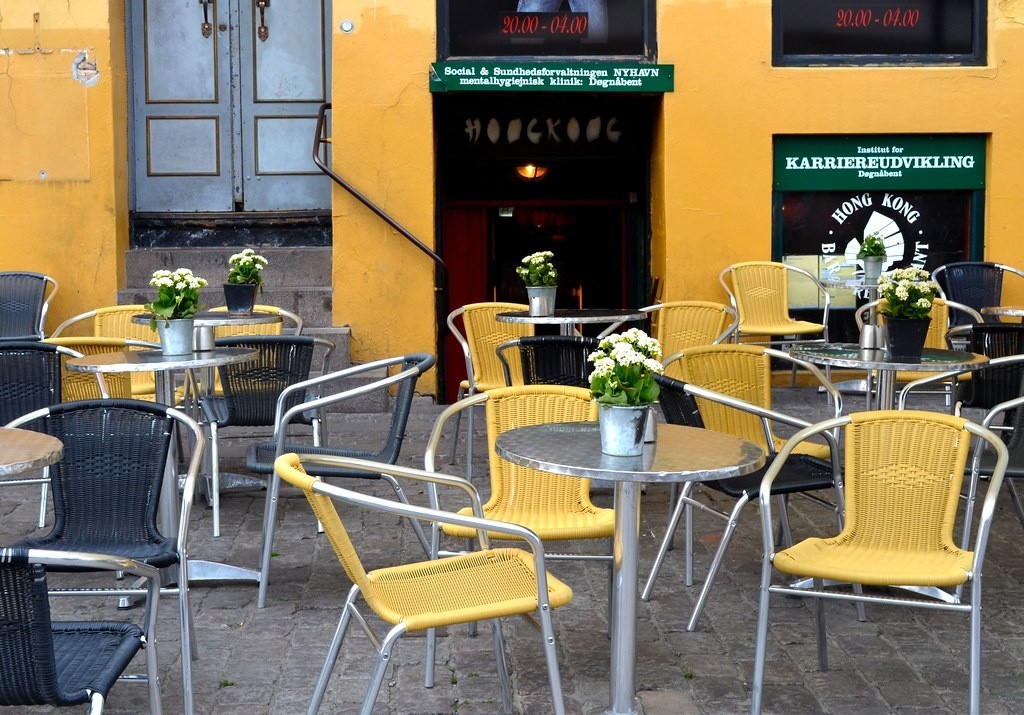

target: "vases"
[155,319,194,356]
[223,283,258,315]
[596,402,650,455]
[860,256,884,279]
[886,315,934,365]
[527,289,556,316]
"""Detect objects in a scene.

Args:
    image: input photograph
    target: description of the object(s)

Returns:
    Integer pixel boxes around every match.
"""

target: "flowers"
[517,251,559,288]
[586,331,661,406]
[228,248,266,286]
[146,268,206,320]
[876,269,938,313]
[856,231,887,256]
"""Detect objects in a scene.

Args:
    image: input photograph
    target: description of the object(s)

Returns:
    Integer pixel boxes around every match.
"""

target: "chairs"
[0,259,1024,715]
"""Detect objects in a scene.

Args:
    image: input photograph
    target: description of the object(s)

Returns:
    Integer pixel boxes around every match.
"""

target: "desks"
[135,309,286,513]
[498,423,768,715]
[0,426,64,476]
[65,347,258,611]
[498,312,651,493]
[816,280,930,404]
[787,343,990,600]
